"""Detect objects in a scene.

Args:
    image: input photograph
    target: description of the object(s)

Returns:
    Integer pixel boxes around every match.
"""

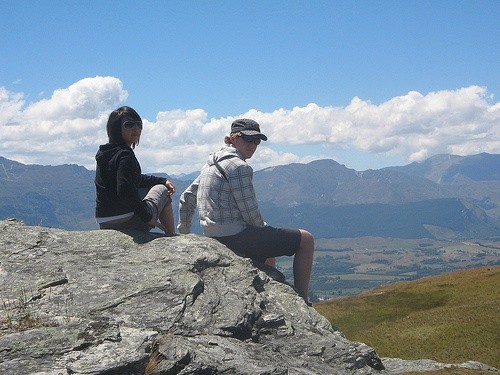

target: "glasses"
[121,120,143,129]
[237,134,262,145]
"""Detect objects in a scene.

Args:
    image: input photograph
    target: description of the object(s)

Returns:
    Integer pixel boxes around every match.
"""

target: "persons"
[91,105,175,239]
[175,119,315,307]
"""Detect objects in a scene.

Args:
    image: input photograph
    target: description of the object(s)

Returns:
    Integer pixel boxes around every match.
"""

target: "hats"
[231,118,268,142]
